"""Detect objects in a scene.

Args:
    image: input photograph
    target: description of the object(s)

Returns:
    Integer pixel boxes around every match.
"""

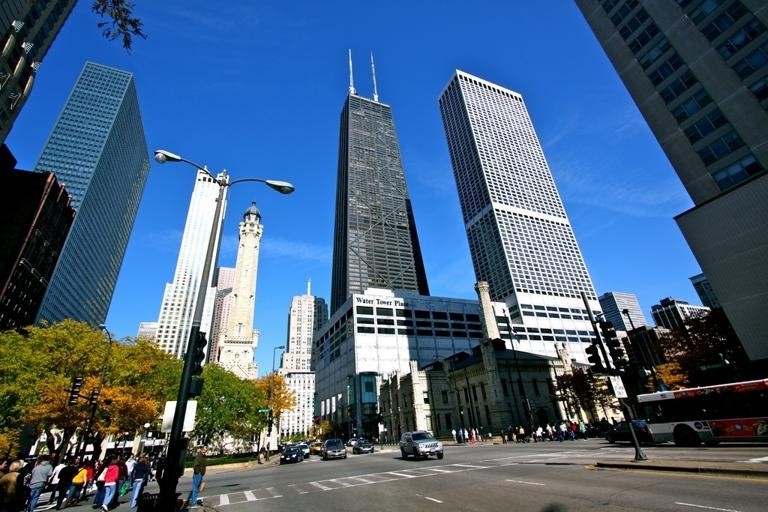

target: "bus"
[636,378,768,447]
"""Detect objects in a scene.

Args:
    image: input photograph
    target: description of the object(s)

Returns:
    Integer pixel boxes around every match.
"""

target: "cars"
[353,437,374,454]
[278,439,324,458]
[280,446,304,464]
[320,439,347,461]
[347,438,357,446]
[605,419,661,445]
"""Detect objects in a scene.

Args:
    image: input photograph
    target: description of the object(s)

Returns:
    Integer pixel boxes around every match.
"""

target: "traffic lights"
[68,376,84,407]
[585,345,603,374]
[600,319,626,371]
[88,388,99,407]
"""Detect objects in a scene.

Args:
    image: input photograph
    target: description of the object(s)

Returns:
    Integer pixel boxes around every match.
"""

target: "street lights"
[122,432,129,457]
[141,423,150,454]
[433,355,466,443]
[266,346,285,461]
[153,150,295,512]
[79,324,112,467]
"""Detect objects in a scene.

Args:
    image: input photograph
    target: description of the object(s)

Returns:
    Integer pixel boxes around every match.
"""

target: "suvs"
[399,430,443,461]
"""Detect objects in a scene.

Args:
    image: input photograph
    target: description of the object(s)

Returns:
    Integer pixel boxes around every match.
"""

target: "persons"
[501,416,624,442]
[0,444,207,511]
[452,425,486,445]
